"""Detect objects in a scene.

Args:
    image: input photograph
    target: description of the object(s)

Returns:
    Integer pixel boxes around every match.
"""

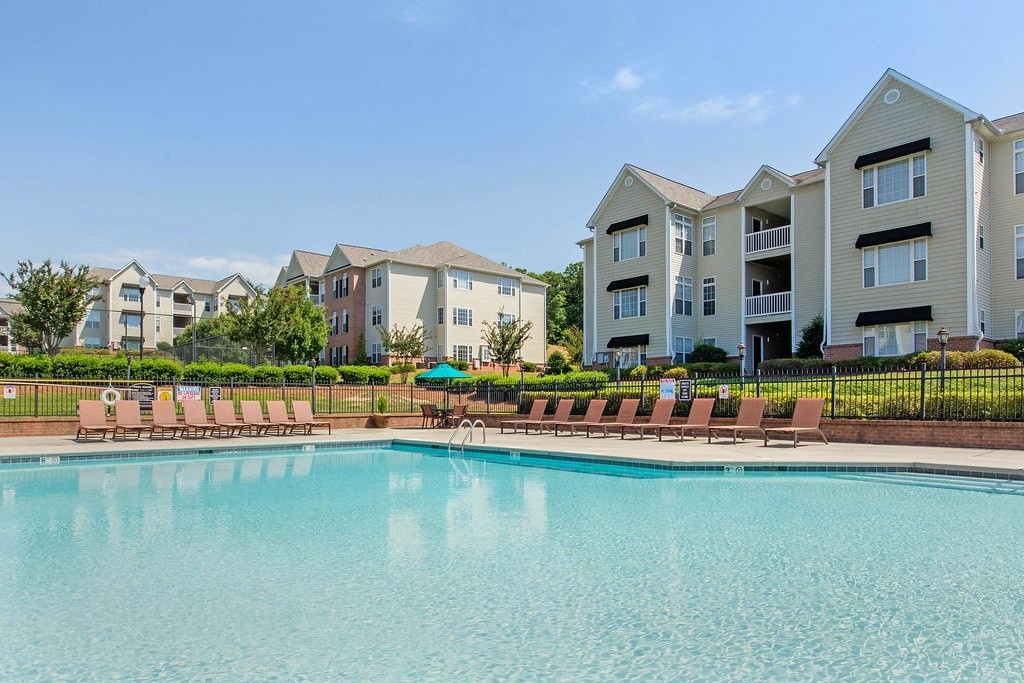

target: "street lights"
[310,357,316,414]
[736,342,746,390]
[186,294,198,365]
[138,275,148,360]
[936,324,950,393]
[616,350,622,391]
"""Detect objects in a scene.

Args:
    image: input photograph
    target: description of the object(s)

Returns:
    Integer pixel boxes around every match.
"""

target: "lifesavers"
[102,389,121,406]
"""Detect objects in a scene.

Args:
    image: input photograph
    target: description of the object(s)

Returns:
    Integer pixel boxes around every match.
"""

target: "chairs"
[183,401,220,440]
[112,400,152,443]
[265,400,306,437]
[706,398,768,444]
[240,399,280,438]
[152,399,190,440]
[525,398,577,437]
[445,405,469,428]
[421,403,442,428]
[585,399,641,439]
[553,400,609,437]
[291,400,333,437]
[213,398,253,439]
[657,398,718,443]
[763,396,828,448]
[499,400,549,434]
[620,398,679,440]
[76,399,116,444]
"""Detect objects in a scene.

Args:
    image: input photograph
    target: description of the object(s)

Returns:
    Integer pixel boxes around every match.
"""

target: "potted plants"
[373,394,391,428]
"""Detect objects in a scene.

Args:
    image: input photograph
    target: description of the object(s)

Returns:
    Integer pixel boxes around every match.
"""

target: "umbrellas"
[416,364,472,428]
